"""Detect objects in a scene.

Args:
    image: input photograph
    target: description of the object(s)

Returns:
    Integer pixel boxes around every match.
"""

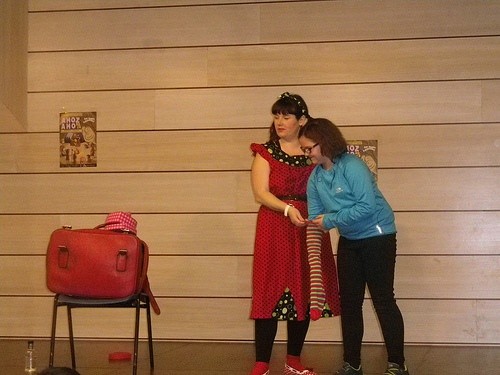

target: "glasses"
[300,142,320,153]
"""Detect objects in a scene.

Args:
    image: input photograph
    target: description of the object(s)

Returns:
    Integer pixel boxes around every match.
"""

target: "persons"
[298,118,409,375]
[250,90,342,375]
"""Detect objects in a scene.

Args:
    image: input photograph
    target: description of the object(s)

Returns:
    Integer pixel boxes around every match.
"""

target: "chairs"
[48,223,155,375]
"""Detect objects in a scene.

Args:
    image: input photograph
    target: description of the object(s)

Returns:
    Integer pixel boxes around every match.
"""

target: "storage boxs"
[105,212,137,235]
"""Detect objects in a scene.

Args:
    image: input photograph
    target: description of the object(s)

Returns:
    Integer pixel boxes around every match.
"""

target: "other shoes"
[336,362,363,375]
[283,361,318,375]
[384,362,409,375]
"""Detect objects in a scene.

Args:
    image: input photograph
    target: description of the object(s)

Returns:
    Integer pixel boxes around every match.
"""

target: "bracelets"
[284,205,290,216]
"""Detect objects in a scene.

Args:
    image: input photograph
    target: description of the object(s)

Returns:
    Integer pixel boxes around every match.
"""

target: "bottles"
[25,340,38,372]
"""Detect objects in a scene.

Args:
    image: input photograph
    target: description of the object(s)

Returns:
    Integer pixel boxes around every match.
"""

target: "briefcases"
[45,221,149,299]
